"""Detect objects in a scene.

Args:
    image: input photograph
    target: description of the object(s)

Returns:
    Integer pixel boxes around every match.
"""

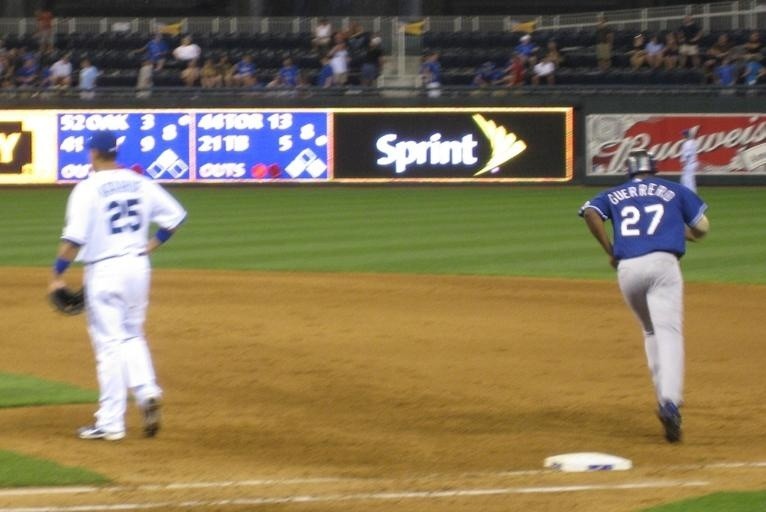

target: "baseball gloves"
[49,287,84,315]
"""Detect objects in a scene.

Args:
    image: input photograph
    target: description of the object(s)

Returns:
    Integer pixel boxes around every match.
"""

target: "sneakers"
[658,402,680,441]
[77,425,126,440]
[141,395,160,438]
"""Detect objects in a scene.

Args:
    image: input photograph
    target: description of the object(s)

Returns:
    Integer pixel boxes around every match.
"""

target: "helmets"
[628,149,653,176]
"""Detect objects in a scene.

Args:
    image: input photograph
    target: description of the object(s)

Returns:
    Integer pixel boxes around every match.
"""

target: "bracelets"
[156,229,172,242]
[53,257,70,273]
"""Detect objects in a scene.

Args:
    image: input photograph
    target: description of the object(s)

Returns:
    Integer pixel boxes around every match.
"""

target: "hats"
[82,134,116,152]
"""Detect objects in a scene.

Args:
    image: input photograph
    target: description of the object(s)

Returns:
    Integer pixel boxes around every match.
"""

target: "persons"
[577,151,712,442]
[1,17,385,100]
[423,12,766,100]
[45,131,189,441]
[678,129,699,195]
